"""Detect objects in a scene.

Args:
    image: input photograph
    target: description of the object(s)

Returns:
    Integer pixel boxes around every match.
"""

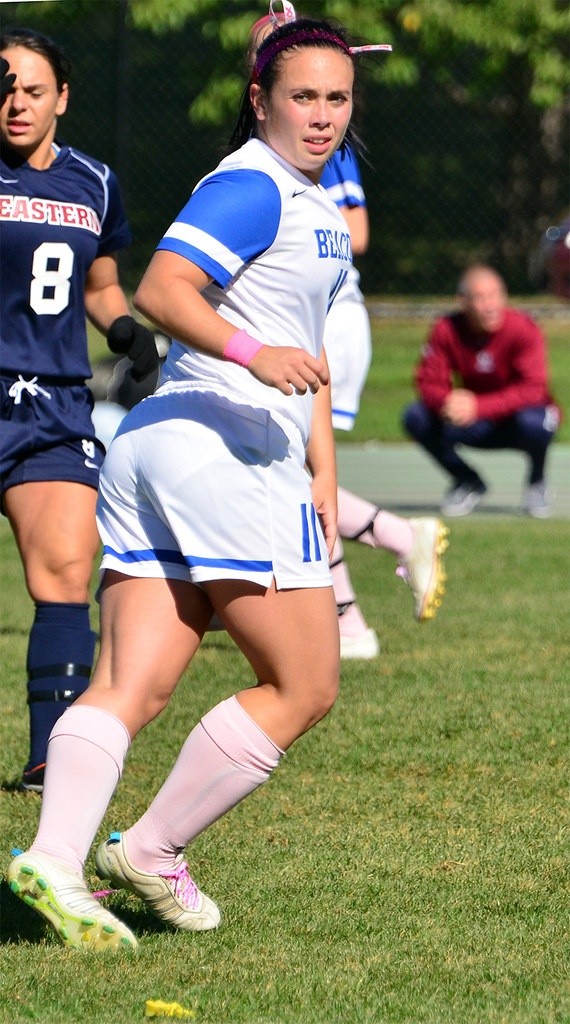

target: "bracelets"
[223,329,264,368]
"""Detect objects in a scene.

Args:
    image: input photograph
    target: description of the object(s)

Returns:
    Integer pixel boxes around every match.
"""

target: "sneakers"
[340,628,381,660]
[6,848,139,964]
[95,832,221,931]
[395,517,451,623]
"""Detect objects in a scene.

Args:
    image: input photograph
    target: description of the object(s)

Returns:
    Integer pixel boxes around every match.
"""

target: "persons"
[0,27,159,790]
[245,11,449,659]
[401,264,563,517]
[8,0,352,951]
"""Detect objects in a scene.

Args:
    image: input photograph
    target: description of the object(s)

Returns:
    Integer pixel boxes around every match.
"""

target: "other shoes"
[440,482,486,516]
[21,763,45,792]
[523,482,553,519]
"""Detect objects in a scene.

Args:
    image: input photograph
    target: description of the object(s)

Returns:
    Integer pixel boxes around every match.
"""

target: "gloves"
[0,56,16,109]
[107,316,158,383]
